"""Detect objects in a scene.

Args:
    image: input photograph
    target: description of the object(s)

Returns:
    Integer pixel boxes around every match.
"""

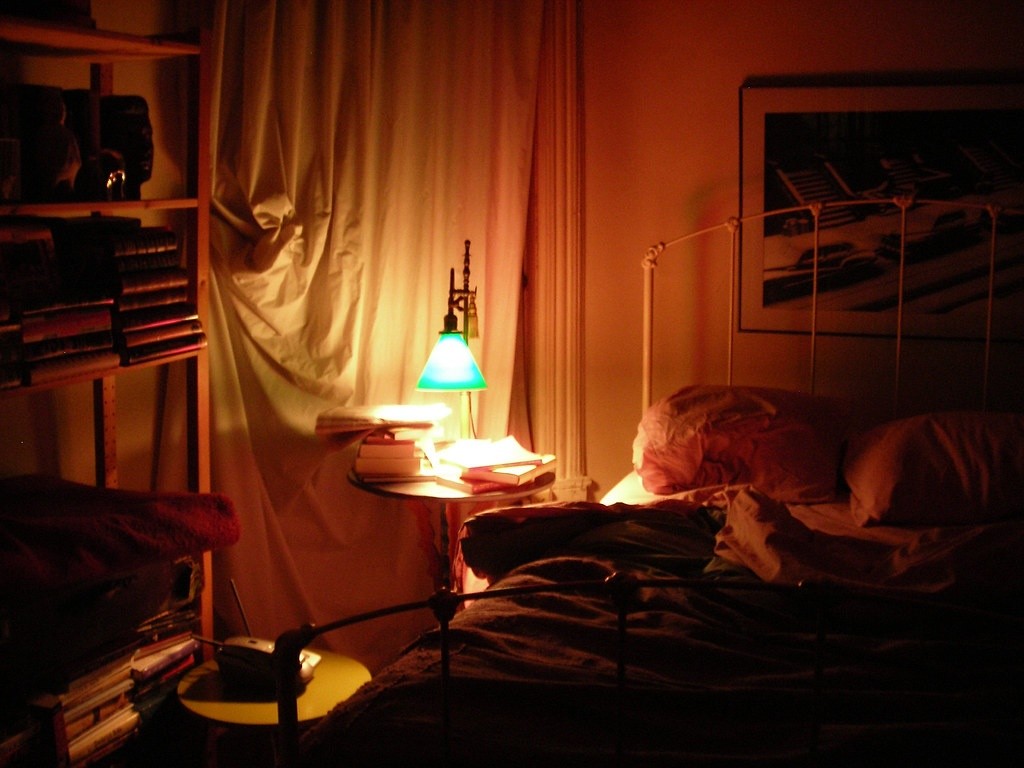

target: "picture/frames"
[738,80,1024,345]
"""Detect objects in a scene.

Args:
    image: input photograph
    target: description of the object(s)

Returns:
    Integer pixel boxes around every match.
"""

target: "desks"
[175,650,373,768]
[346,440,556,592]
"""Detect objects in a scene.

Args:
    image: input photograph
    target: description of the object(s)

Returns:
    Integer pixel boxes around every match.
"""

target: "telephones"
[188,630,321,694]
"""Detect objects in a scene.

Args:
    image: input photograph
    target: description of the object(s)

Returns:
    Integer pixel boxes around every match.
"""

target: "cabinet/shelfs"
[0,16,214,768]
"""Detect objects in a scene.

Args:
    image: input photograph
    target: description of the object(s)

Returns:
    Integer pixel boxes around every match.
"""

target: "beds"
[275,193,1024,767]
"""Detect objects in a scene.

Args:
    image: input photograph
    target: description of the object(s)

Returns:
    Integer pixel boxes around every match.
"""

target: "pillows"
[631,384,850,511]
[841,409,1024,528]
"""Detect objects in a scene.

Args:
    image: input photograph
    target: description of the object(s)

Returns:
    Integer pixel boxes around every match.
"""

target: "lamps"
[415,237,489,438]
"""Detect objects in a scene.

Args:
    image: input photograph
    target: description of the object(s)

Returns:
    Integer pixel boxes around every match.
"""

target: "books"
[0,227,209,384]
[1,630,203,767]
[316,404,558,494]
